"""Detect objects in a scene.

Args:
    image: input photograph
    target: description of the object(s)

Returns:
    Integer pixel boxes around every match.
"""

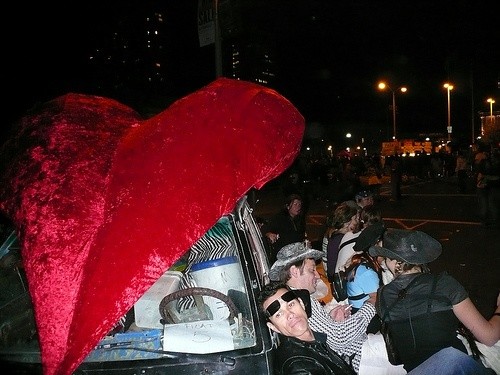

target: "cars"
[0,194,282,375]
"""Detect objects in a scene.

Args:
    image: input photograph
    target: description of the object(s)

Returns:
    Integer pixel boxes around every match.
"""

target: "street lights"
[443,84,453,141]
[487,98,495,126]
[378,82,407,142]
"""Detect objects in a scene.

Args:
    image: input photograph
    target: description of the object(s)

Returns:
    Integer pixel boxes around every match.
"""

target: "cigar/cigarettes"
[345,304,353,310]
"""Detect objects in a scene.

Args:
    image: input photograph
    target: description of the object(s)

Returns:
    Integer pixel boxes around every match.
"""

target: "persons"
[257,138,500,375]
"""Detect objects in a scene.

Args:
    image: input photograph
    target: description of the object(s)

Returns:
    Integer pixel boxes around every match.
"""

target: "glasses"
[264,291,298,321]
[291,202,301,207]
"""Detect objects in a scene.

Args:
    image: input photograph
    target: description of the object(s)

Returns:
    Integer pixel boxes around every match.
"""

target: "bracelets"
[492,312,500,316]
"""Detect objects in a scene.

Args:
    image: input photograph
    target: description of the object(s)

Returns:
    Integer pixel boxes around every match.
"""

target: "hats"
[353,182,371,197]
[353,222,386,250]
[368,227,442,264]
[269,242,325,281]
